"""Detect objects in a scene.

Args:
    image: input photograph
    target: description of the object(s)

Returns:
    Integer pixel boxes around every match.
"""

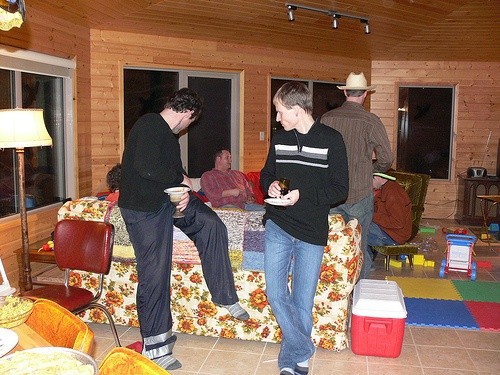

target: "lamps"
[332,15,339,29]
[0,107,52,291]
[287,5,295,21]
[0,0,26,31]
[365,20,370,33]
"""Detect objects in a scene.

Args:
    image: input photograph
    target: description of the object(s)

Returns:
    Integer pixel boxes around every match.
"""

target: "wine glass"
[163,186,190,219]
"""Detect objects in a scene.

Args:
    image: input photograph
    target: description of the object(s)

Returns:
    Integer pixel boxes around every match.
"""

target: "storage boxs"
[352,277,410,359]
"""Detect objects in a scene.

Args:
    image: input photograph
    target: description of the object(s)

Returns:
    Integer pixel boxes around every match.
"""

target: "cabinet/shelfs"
[456,174,500,226]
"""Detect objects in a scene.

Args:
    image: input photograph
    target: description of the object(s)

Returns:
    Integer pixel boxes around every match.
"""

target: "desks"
[476,195,500,249]
[14,235,56,294]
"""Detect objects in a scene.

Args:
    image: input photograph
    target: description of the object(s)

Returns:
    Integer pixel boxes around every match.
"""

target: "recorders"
[467,167,487,178]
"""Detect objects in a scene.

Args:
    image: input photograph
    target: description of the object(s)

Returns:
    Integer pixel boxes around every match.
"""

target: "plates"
[0,346,98,375]
[264,198,294,206]
[0,326,19,358]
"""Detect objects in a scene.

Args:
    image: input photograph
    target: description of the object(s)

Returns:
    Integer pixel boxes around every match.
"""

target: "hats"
[337,71,376,92]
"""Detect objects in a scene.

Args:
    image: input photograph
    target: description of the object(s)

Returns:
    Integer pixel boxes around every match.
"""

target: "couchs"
[57,195,364,352]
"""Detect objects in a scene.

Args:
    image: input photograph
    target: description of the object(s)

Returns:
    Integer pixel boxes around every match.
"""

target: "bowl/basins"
[0,295,34,329]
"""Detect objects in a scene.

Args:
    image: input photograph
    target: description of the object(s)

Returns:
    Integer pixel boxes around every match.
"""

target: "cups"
[278,177,289,198]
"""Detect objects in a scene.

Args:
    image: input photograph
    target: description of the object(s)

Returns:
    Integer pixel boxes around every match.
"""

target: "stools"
[372,244,418,271]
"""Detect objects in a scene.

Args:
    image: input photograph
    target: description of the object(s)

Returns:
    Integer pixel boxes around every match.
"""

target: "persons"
[104,164,120,202]
[118,87,249,370]
[320,71,392,284]
[366,168,413,271]
[200,148,266,211]
[259,81,350,375]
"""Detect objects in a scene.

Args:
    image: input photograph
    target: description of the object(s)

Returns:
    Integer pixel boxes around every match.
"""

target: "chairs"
[384,169,431,242]
[99,347,170,375]
[25,298,95,354]
[18,218,122,346]
